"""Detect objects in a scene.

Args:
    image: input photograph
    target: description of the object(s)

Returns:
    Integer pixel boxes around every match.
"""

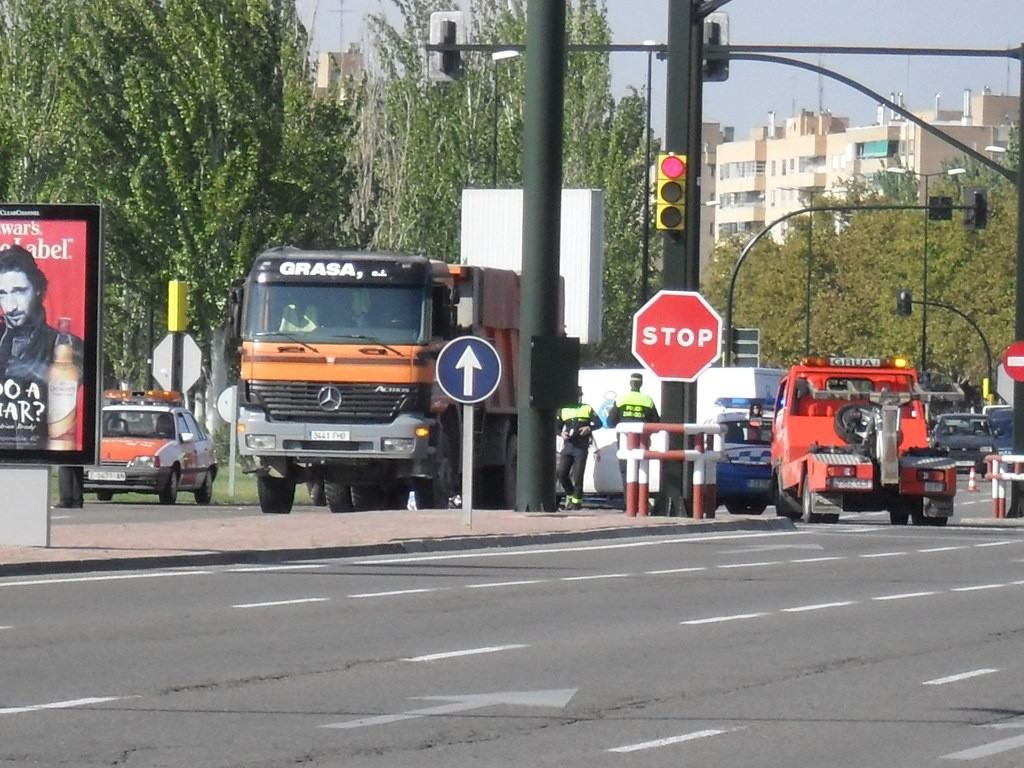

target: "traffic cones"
[968,466,978,492]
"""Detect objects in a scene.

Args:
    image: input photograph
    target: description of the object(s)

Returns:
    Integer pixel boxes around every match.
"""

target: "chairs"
[726,425,744,443]
[973,422,984,434]
[946,425,957,435]
[107,418,128,434]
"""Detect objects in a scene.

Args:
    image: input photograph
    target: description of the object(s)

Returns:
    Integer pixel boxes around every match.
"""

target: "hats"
[577,386,583,397]
[630,373,642,382]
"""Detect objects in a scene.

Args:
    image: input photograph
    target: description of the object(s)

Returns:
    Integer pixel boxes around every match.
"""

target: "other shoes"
[567,501,580,510]
[55,503,69,508]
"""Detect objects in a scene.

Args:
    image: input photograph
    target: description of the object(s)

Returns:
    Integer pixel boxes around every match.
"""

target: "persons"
[556,387,602,510]
[606,374,661,514]
[278,287,319,333]
[145,414,175,440]
[0,243,83,449]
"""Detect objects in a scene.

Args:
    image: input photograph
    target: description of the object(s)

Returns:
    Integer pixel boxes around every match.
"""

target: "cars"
[931,406,1015,474]
[704,396,784,514]
[83,389,219,505]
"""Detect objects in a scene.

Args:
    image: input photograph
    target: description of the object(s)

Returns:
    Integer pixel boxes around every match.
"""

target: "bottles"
[47,317,80,449]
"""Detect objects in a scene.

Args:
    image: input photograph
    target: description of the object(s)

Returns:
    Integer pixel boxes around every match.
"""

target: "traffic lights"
[657,155,687,230]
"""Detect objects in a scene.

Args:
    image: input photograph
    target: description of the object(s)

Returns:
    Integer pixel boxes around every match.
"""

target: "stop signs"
[1003,341,1024,382]
[632,290,723,383]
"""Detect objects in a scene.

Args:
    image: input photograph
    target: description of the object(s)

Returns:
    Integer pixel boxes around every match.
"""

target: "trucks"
[228,245,567,513]
[565,379,568,381]
[770,356,957,526]
